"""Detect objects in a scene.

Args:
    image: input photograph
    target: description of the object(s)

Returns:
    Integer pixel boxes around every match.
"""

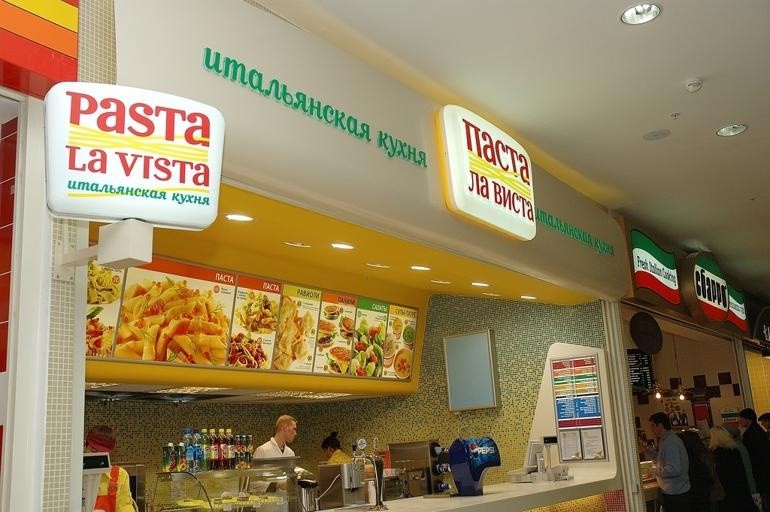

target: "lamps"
[672,334,688,400]
[650,354,664,400]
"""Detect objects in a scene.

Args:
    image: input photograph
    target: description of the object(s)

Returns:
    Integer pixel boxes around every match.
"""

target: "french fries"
[113,276,229,368]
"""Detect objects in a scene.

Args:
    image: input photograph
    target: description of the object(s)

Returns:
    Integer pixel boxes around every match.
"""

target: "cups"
[436,464,452,491]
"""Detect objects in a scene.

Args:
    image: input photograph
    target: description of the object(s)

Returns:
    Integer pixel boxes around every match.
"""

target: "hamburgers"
[317,305,355,376]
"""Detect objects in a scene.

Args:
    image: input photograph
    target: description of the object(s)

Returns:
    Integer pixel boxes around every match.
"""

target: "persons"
[82,425,137,512]
[320,431,352,465]
[675,429,713,512]
[738,407,770,512]
[248,414,299,511]
[709,424,759,512]
[757,412,770,434]
[720,423,757,498]
[637,411,692,511]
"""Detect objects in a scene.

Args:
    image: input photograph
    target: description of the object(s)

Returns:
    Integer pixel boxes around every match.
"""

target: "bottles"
[184,428,254,474]
[668,409,688,426]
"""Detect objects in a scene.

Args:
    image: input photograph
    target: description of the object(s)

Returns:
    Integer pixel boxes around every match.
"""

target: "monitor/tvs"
[523,440,544,468]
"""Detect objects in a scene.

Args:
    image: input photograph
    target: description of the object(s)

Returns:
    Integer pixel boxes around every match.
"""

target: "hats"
[86,424,117,452]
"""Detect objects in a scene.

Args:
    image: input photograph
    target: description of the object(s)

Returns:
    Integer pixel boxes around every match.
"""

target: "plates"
[176,495,278,509]
